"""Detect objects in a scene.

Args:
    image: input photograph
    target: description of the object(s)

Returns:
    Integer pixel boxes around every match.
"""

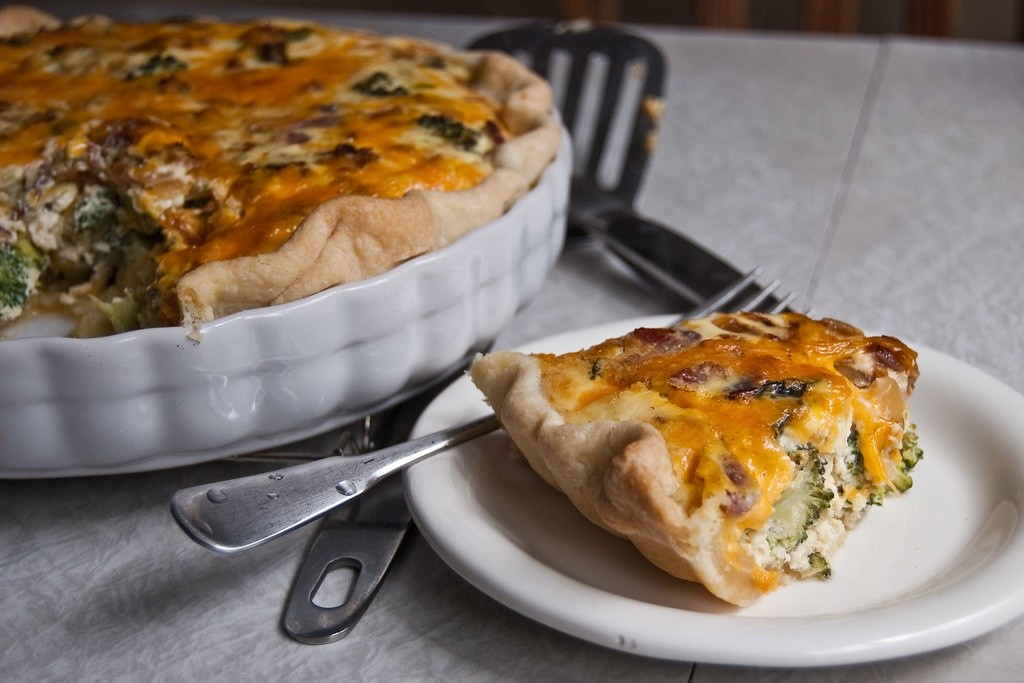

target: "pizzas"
[0,1,558,331]
[466,308,917,605]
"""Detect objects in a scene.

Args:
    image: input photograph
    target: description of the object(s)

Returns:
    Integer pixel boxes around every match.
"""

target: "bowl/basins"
[0,107,571,478]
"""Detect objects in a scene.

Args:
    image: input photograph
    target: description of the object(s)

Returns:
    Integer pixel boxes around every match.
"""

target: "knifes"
[569,180,803,313]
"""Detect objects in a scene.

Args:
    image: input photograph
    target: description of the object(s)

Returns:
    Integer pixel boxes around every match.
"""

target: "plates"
[402,314,1024,668]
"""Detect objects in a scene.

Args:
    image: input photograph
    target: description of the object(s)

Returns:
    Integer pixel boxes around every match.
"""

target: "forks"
[165,268,814,555]
